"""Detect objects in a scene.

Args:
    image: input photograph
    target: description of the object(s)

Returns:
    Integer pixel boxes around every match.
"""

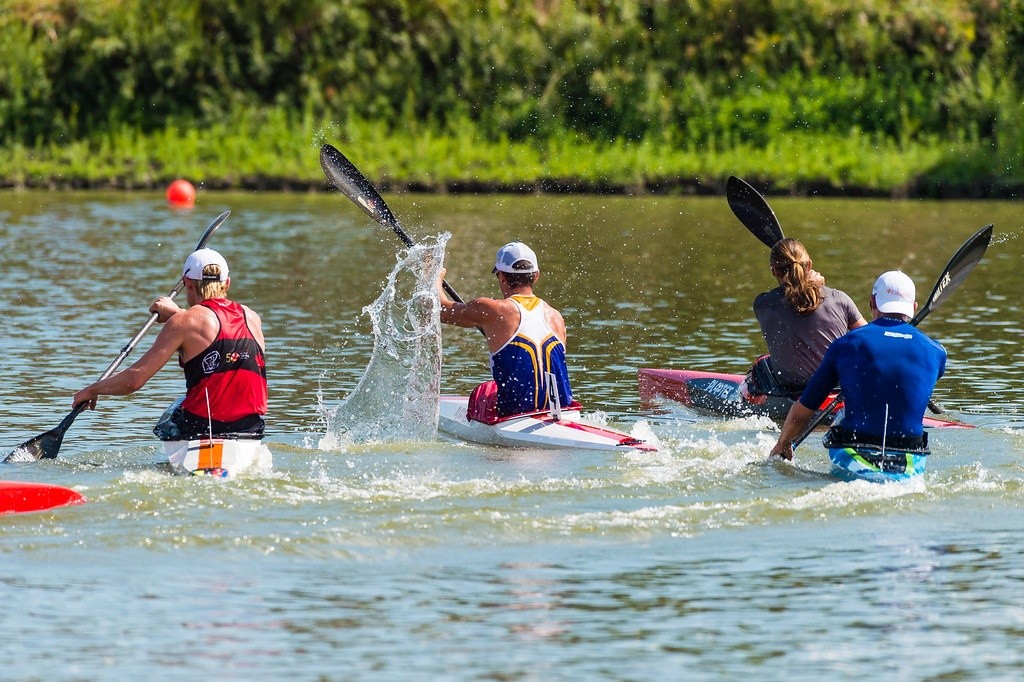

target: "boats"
[425,396,660,452]
[162,438,260,483]
[828,407,927,486]
[638,368,978,432]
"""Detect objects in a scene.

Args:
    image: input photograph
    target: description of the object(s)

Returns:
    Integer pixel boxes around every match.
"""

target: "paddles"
[782,223,994,459]
[319,143,486,336]
[1,209,233,464]
[726,175,944,414]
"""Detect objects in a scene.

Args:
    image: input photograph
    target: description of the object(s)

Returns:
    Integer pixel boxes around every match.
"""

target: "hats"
[872,270,916,319]
[182,248,229,282]
[491,241,539,273]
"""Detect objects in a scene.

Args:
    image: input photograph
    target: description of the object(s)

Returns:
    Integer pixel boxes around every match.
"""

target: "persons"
[770,270,947,473]
[437,241,573,417]
[72,247,268,440]
[752,238,868,397]
